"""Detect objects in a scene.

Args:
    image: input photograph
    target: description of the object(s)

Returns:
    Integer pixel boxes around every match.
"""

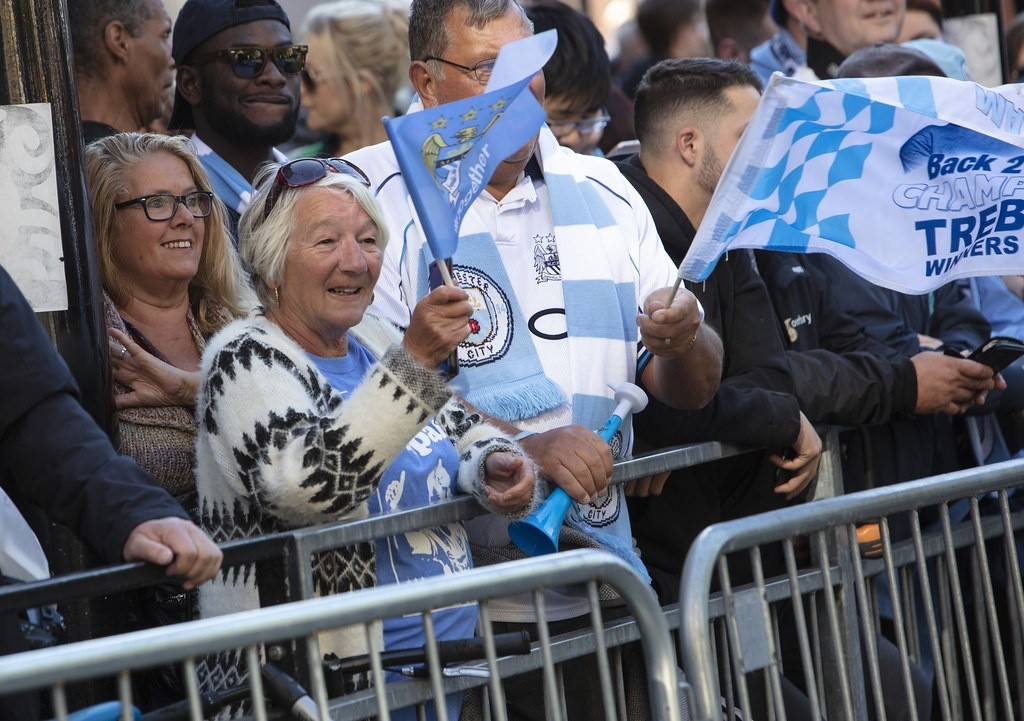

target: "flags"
[679,72,1024,297]
[384,29,561,260]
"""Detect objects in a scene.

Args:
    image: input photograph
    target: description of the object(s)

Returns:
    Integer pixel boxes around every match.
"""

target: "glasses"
[547,105,610,136]
[254,157,371,229]
[424,55,497,81]
[187,45,308,78]
[303,70,327,93]
[114,190,214,221]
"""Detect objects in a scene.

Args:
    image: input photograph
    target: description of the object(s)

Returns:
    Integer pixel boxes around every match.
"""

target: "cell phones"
[969,335,1024,379]
[605,139,641,158]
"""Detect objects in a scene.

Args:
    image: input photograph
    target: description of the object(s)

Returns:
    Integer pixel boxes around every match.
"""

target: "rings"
[119,346,128,362]
[666,338,670,349]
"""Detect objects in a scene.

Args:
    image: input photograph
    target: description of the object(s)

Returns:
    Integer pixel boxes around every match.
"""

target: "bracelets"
[685,332,698,353]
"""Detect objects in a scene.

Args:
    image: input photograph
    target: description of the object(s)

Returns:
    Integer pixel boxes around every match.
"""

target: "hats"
[171,0,290,62]
[902,39,969,81]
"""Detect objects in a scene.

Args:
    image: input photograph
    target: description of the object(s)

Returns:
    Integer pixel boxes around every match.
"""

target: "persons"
[167,0,311,251]
[194,158,538,721]
[80,132,259,721]
[805,252,1024,720]
[0,263,224,721]
[280,0,416,162]
[519,1,1024,154]
[610,57,824,721]
[67,0,176,155]
[322,0,725,721]
